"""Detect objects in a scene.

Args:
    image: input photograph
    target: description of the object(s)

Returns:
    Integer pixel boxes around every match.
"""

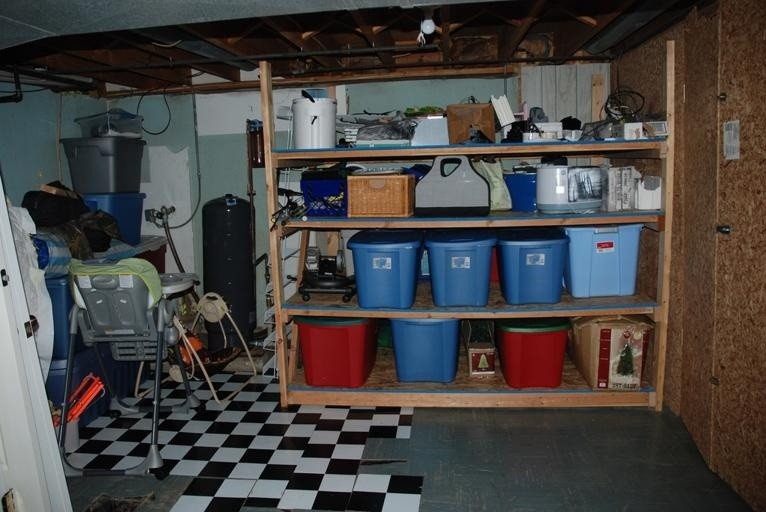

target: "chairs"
[54,258,200,476]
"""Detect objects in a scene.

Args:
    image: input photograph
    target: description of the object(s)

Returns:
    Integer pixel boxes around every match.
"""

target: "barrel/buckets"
[291,91,336,152]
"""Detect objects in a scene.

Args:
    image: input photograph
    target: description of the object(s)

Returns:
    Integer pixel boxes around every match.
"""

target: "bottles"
[249,119,266,168]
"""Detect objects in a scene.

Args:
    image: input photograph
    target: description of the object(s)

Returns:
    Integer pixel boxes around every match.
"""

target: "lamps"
[418,7,439,36]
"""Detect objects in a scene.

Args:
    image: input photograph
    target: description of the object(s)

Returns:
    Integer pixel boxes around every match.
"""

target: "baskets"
[447,104,497,144]
[346,173,416,219]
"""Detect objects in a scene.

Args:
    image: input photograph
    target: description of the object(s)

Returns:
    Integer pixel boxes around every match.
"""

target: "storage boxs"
[386,317,460,384]
[44,107,166,428]
[555,224,644,299]
[567,314,654,391]
[291,315,380,388]
[493,228,570,305]
[493,319,568,390]
[344,230,423,310]
[422,230,498,307]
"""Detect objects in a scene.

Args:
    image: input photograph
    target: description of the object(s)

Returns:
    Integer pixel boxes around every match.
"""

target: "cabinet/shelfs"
[270,137,668,407]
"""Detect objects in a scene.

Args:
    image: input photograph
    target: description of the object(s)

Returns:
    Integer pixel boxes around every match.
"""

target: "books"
[488,93,517,139]
